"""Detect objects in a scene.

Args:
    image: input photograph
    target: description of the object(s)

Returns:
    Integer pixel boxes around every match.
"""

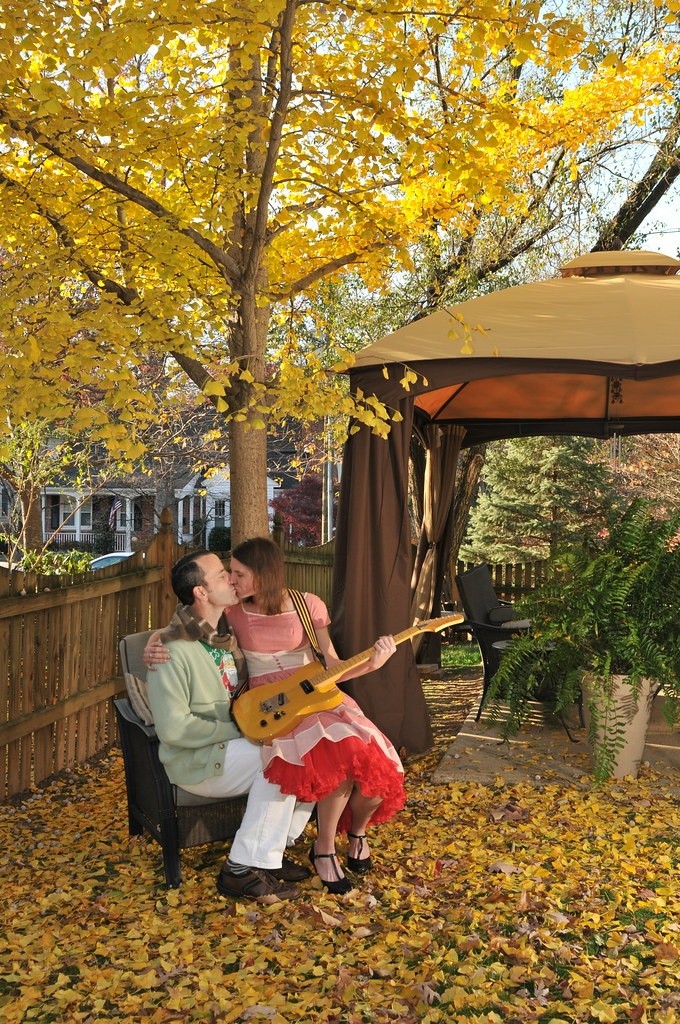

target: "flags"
[108,496,122,528]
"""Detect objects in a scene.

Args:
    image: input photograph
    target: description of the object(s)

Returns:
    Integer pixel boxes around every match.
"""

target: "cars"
[86,551,146,570]
[0,515,24,553]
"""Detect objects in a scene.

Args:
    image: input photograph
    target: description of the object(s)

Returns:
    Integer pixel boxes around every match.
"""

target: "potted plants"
[480,489,680,787]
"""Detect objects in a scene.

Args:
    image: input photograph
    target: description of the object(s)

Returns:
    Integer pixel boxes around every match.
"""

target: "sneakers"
[215,854,303,901]
[266,857,311,881]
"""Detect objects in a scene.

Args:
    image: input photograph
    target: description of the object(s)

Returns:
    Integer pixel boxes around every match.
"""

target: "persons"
[142,537,407,893]
[146,550,316,900]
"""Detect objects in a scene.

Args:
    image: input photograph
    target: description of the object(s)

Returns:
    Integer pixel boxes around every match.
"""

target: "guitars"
[231,611,465,745]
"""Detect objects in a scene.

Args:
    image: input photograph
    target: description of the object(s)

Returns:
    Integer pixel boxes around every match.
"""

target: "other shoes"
[348,828,373,874]
[308,840,352,894]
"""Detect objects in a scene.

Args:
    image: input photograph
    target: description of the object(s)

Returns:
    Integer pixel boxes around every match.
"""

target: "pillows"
[125,673,154,726]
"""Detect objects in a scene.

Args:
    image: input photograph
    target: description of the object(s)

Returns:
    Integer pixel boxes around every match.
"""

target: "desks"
[492,640,580,745]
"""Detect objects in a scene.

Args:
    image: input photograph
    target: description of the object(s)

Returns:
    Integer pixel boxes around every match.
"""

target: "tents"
[329,252,680,757]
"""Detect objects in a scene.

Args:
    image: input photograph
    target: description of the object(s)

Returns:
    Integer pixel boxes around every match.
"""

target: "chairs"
[114,628,316,889]
[444,602,473,646]
[455,562,585,728]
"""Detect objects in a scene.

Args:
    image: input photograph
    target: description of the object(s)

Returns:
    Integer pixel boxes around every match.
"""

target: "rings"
[149,653,151,658]
